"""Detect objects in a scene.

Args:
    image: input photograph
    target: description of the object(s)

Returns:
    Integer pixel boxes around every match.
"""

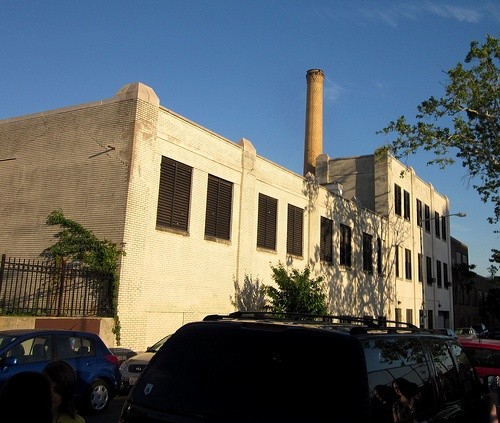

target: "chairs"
[5,344,28,367]
[76,346,90,355]
[28,344,47,362]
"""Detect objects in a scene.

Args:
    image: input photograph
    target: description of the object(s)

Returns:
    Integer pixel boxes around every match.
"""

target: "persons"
[0,359,88,423]
[373,384,400,415]
[390,377,436,423]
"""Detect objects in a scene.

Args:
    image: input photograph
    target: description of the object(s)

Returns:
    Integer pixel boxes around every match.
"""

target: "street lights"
[419,211,467,329]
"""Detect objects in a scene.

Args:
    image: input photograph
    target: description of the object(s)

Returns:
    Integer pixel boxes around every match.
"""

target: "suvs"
[116,311,500,423]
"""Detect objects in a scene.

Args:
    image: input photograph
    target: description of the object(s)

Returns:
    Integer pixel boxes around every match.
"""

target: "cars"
[454,327,478,340]
[437,327,456,336]
[455,338,500,391]
[0,330,122,415]
[118,336,171,395]
[470,323,489,339]
